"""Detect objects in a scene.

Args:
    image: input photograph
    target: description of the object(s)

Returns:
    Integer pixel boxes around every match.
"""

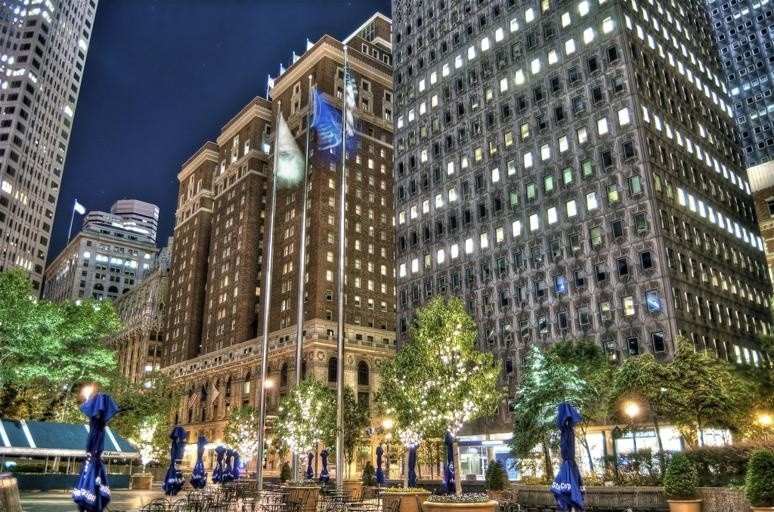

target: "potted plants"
[743,447,774,512]
[664,449,703,512]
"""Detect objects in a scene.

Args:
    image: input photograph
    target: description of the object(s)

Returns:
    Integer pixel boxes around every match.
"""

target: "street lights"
[758,415,771,447]
[620,398,644,463]
[80,382,98,425]
[381,419,394,482]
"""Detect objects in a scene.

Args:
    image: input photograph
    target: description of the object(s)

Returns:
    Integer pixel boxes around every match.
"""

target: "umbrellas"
[162,426,189,512]
[374,447,386,488]
[222,449,234,486]
[71,392,117,512]
[306,452,314,484]
[320,450,330,486]
[190,436,208,498]
[213,446,224,489]
[549,403,588,512]
[233,451,240,481]
[407,446,418,489]
[442,431,457,495]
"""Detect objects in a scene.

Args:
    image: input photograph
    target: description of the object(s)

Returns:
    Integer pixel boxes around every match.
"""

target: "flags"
[343,59,358,158]
[276,111,306,185]
[311,84,342,160]
[74,201,86,215]
